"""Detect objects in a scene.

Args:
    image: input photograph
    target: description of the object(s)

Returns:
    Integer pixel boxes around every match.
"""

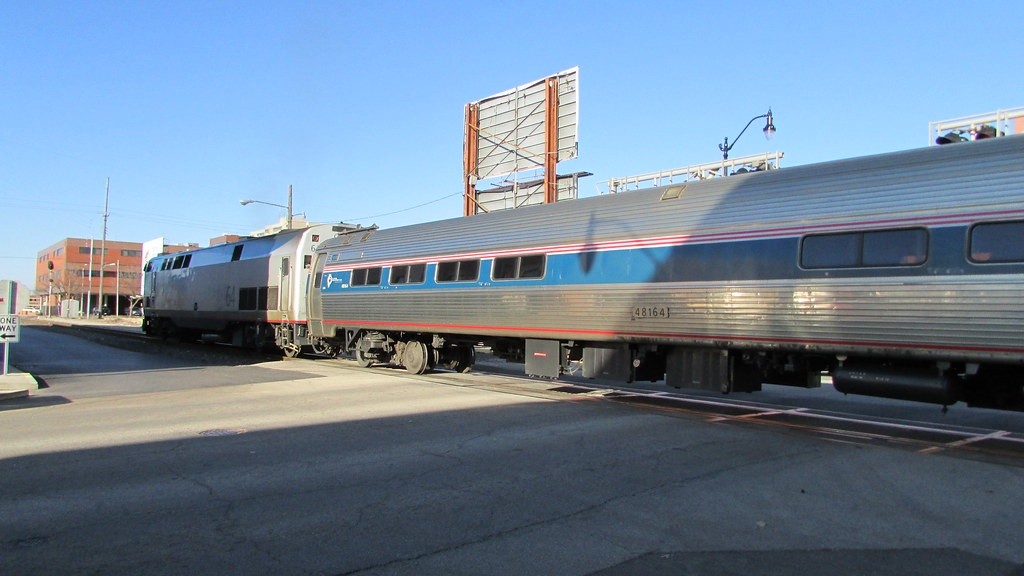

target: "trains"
[141,133,1024,415]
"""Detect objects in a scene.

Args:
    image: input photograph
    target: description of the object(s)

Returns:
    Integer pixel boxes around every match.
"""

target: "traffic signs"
[0,315,20,343]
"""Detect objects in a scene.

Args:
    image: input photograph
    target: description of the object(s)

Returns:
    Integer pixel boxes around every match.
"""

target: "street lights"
[240,199,307,228]
[718,105,777,178]
